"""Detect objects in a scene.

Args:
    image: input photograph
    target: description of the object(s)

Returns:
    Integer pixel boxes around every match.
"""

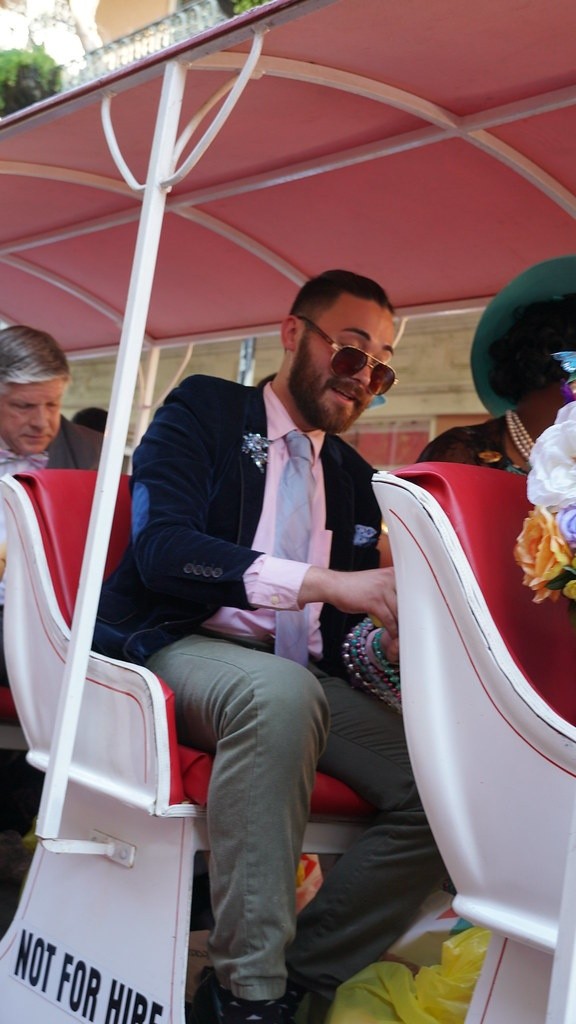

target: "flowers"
[511,352,576,607]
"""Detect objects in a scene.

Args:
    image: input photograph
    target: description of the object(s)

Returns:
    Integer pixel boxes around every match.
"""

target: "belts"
[193,627,274,655]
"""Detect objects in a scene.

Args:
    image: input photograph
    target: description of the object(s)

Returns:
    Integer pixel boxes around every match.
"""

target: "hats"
[471,255,576,421]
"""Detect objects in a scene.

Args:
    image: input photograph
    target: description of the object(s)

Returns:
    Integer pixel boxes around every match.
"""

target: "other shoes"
[0,830,33,885]
[189,965,296,1024]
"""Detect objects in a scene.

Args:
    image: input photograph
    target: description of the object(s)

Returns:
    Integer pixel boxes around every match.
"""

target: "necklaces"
[506,409,535,460]
[343,617,401,709]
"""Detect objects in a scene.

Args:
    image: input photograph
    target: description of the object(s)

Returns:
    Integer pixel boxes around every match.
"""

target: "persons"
[0,326,104,689]
[93,270,445,1024]
[419,254,576,478]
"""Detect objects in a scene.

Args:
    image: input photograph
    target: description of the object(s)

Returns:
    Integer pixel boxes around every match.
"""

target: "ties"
[274,430,312,672]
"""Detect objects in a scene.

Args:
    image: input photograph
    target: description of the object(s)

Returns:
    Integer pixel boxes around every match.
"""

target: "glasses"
[296,314,399,396]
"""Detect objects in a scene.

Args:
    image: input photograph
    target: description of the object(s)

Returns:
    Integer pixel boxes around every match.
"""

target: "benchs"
[0,469,362,855]
[371,461,576,954]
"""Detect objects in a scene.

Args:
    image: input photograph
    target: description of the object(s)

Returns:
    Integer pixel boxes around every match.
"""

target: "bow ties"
[0,449,50,469]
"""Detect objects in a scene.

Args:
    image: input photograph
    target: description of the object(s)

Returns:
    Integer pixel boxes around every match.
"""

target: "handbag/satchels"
[323,927,492,1024]
